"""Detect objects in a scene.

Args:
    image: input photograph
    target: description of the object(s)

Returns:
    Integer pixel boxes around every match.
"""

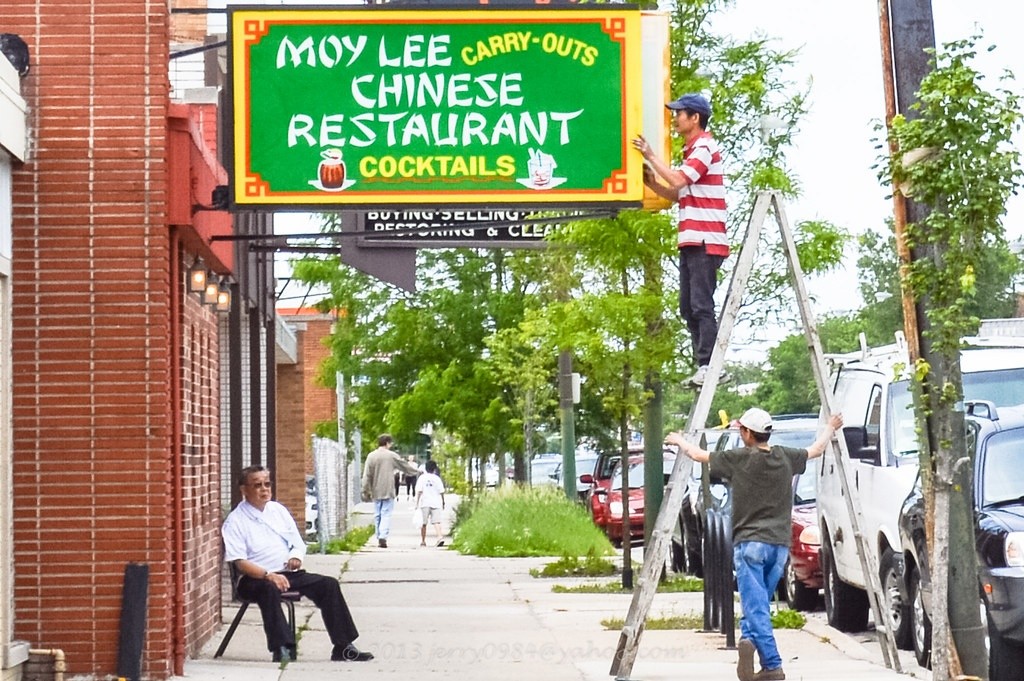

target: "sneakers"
[331,642,374,662]
[737,637,756,680]
[682,363,730,393]
[751,666,785,681]
[272,646,292,662]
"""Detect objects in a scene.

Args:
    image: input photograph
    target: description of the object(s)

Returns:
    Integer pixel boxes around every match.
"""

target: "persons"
[221,466,375,662]
[361,434,423,548]
[394,455,441,502]
[631,94,731,390]
[664,407,844,681]
[415,460,445,546]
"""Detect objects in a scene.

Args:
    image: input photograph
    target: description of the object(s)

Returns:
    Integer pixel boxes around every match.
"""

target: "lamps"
[201,269,218,304]
[186,253,207,292]
[213,279,231,313]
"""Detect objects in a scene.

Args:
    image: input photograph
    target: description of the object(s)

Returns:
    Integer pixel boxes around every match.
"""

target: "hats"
[664,94,711,117]
[734,408,774,433]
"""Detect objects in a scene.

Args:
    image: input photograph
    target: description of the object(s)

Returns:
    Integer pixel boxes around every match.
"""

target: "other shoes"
[437,540,444,546]
[421,542,426,547]
[378,537,388,548]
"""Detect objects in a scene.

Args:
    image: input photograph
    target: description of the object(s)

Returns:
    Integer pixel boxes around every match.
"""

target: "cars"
[547,455,598,499]
[594,453,676,549]
[660,419,824,611]
[579,447,677,533]
[486,471,499,486]
[530,459,560,484]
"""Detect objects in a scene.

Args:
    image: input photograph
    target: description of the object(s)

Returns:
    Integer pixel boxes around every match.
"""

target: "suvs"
[898,400,1024,681]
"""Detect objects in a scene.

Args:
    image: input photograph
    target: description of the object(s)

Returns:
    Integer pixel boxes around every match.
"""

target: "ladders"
[608,190,902,681]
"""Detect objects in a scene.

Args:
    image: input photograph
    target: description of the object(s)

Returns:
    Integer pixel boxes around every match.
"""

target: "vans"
[816,336,1024,651]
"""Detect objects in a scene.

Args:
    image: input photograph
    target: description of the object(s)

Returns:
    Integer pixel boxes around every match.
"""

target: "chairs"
[214,541,304,660]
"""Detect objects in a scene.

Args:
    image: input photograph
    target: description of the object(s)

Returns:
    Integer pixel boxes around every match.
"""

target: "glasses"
[245,482,271,488]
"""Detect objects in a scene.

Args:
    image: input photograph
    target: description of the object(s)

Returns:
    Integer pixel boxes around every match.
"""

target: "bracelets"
[264,571,270,580]
